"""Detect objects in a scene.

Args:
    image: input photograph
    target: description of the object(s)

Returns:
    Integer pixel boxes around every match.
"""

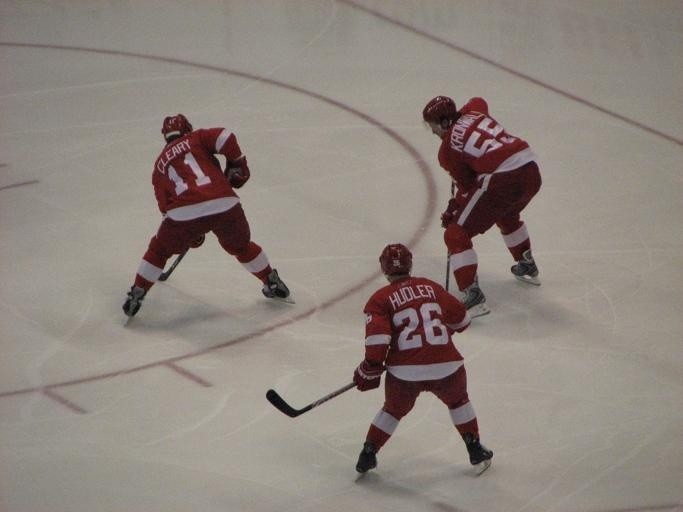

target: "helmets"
[161,113,192,141]
[379,243,413,276]
[422,95,457,129]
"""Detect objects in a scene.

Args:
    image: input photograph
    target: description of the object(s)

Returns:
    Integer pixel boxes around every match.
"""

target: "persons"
[123,114,290,315]
[423,96,542,309]
[352,244,492,473]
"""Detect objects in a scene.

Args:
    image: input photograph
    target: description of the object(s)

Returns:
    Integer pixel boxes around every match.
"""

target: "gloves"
[224,154,250,189]
[353,358,382,392]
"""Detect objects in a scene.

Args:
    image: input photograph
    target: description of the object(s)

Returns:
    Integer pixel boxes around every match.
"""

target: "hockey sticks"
[266,366,386,418]
[159,169,241,281]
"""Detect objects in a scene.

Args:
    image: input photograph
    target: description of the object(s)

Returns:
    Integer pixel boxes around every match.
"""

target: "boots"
[457,274,485,311]
[355,440,380,474]
[510,248,540,280]
[261,268,290,299]
[461,433,494,465]
[121,284,147,318]
